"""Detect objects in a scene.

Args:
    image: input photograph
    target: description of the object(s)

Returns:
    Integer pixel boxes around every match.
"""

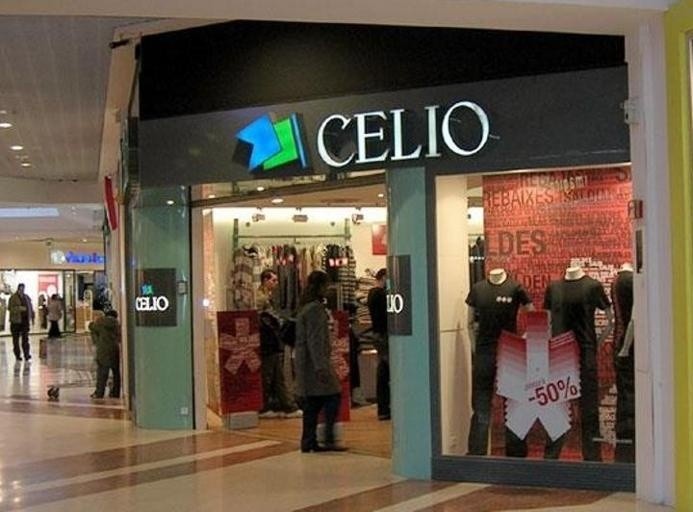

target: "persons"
[610,261,634,462]
[367,268,391,419]
[89,309,121,402]
[465,268,536,459]
[8,283,35,361]
[255,269,304,419]
[47,295,62,337]
[543,267,613,462]
[293,269,372,452]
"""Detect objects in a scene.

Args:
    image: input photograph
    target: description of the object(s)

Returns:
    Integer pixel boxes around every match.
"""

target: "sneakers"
[257,401,304,419]
[109,391,120,398]
[377,411,392,421]
[89,392,106,399]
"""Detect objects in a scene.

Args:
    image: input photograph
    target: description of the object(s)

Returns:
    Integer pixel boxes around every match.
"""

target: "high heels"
[298,436,351,453]
